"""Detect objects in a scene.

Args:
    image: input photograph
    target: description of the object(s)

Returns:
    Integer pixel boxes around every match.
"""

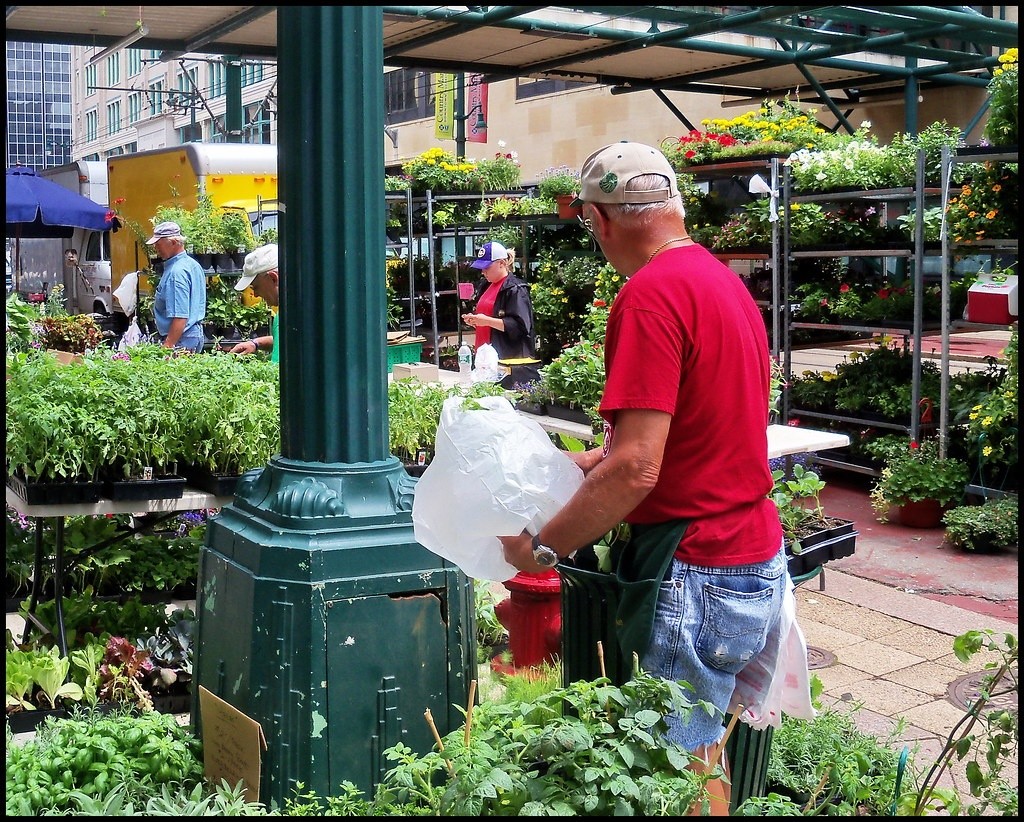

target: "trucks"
[11,159,113,319]
[107,143,279,342]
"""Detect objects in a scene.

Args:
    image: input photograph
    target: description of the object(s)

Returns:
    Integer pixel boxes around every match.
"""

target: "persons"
[146,222,206,354]
[463,242,535,361]
[494,140,788,816]
[227,242,278,363]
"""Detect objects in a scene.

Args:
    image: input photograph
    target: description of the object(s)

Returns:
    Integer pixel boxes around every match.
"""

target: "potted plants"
[7,340,510,505]
[944,497,1020,552]
[148,181,257,269]
[5,285,114,352]
[4,522,1021,820]
[557,428,859,577]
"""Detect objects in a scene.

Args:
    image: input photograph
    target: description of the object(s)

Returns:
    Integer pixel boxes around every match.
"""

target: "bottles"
[457,341,472,384]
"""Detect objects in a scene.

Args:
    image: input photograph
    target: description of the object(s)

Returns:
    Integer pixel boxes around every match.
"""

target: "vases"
[149,257,163,275]
[895,494,951,529]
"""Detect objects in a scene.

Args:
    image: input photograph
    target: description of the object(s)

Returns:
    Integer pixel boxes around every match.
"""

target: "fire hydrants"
[489,570,562,689]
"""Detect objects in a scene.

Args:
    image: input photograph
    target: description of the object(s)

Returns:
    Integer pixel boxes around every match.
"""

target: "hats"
[234,244,278,291]
[569,140,678,207]
[470,242,509,268]
[145,222,180,244]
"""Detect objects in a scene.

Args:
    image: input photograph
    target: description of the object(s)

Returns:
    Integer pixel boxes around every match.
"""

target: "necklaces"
[645,236,692,266]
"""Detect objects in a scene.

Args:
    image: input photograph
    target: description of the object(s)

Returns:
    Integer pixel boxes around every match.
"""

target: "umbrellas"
[6,160,122,293]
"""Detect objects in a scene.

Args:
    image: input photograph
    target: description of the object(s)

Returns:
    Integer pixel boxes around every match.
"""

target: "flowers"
[387,106,1020,591]
[105,197,159,257]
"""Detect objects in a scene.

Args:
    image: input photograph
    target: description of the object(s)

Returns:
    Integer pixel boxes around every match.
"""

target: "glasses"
[576,208,610,232]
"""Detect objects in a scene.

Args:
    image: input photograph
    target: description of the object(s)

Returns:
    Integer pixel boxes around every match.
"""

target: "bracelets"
[248,339,259,350]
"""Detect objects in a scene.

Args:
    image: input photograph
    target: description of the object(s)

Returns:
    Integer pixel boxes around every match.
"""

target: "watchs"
[530,533,561,568]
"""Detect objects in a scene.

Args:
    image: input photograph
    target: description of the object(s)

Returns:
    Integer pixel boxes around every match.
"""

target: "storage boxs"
[966,274,1020,325]
[47,348,84,369]
[384,344,438,382]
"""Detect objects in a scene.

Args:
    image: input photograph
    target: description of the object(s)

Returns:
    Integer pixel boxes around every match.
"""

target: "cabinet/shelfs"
[939,138,1020,506]
[784,149,937,449]
[454,217,584,350]
[410,188,534,368]
[667,158,780,425]
